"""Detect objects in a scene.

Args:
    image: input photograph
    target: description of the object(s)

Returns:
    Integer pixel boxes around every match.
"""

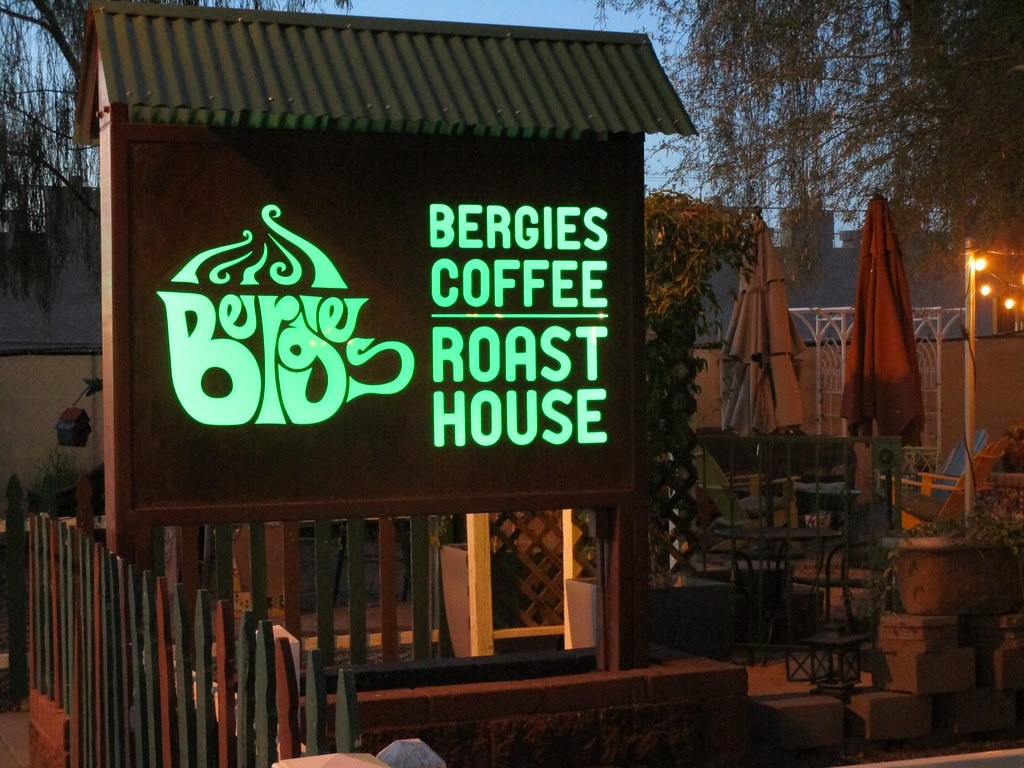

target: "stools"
[901,446,937,473]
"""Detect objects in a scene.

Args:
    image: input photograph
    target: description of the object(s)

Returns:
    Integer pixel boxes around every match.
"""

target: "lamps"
[785,628,869,694]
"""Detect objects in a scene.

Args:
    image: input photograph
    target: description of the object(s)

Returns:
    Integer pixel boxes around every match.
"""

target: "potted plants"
[34,444,81,516]
[567,188,735,655]
[866,508,1023,617]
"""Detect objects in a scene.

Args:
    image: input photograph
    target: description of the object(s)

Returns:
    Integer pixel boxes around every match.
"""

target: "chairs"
[690,444,802,556]
[880,438,1008,530]
[932,429,989,503]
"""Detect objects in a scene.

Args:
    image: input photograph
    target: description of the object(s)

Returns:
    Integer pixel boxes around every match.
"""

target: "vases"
[436,541,564,658]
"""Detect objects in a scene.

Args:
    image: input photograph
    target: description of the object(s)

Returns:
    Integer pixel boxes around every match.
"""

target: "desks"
[804,488,862,525]
[716,527,843,640]
